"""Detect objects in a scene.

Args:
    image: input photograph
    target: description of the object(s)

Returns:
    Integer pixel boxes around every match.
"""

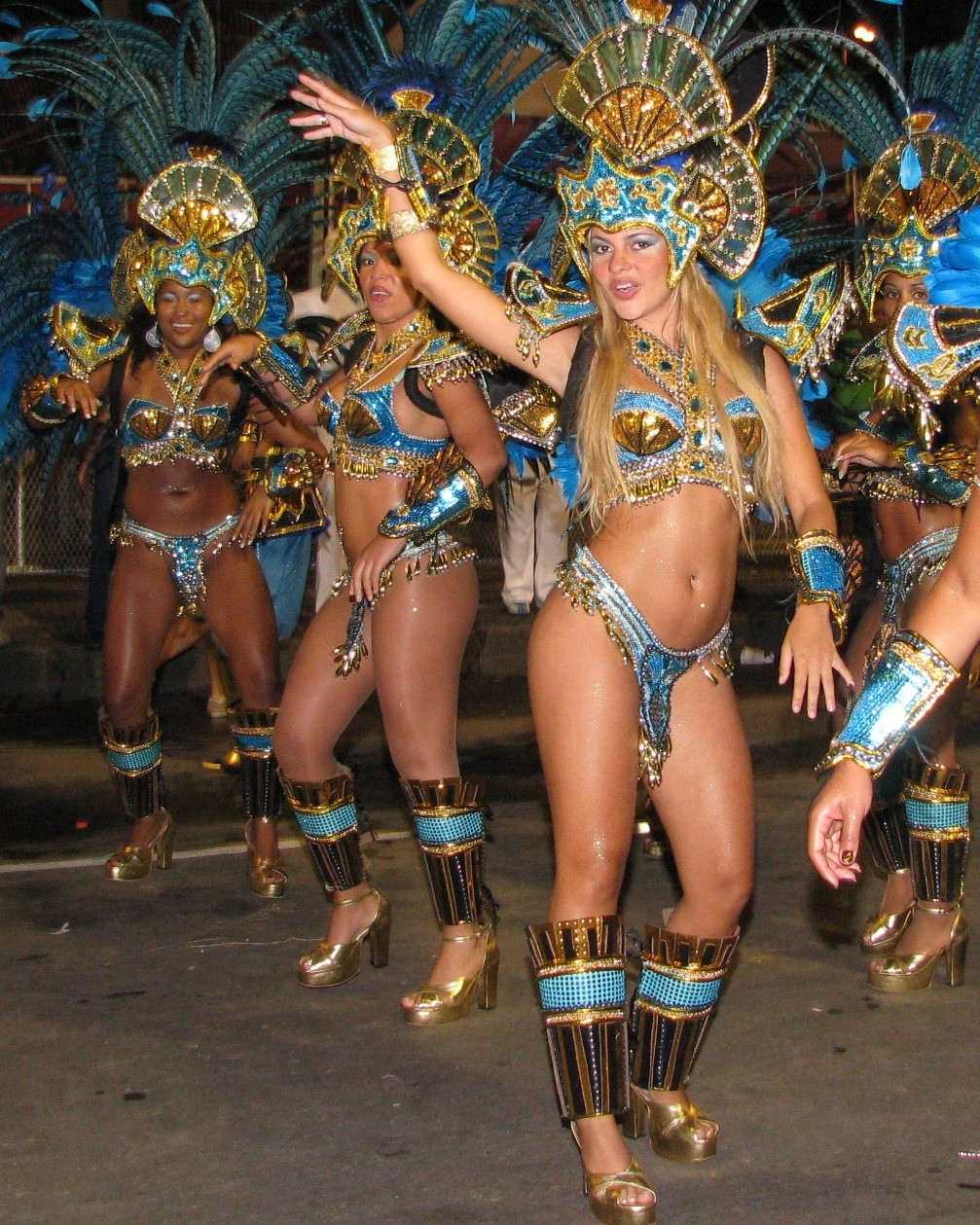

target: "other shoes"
[221,748,241,771]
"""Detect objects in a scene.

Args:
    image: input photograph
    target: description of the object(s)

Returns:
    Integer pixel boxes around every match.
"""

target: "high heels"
[866,900,970,994]
[244,816,288,898]
[622,1084,718,1164]
[402,924,500,1027]
[859,867,916,954]
[298,889,392,987]
[570,1113,657,1225]
[106,806,174,881]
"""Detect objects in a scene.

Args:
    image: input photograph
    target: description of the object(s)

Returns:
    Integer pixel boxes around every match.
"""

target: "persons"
[289,2,855,1225]
[0,0,322,899]
[192,0,510,1026]
[809,0,980,997]
